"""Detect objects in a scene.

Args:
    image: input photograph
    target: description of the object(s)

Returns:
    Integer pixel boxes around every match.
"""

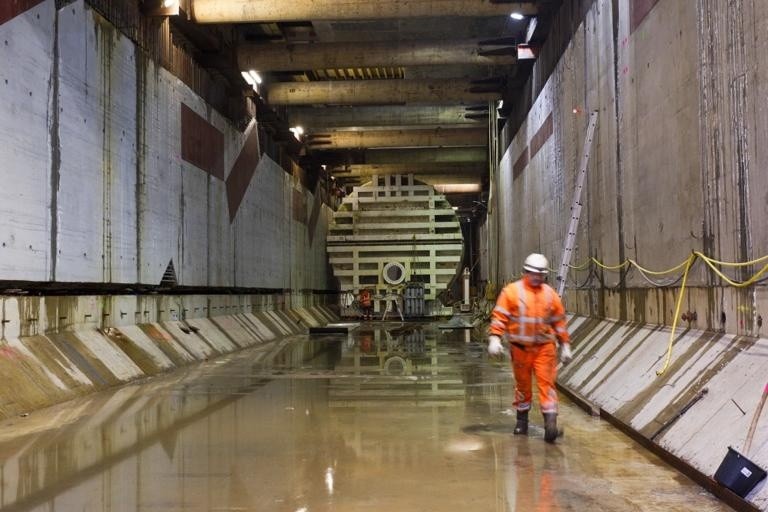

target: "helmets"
[522,253,549,274]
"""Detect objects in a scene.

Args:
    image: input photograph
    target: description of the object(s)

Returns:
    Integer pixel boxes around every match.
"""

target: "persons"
[360,287,372,321]
[513,431,558,511]
[488,253,571,441]
[360,330,373,352]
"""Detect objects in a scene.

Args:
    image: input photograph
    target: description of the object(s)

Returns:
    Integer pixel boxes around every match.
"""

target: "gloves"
[488,335,505,361]
[559,344,572,363]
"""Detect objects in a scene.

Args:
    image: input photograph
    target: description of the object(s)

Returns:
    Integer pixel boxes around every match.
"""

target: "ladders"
[555,109,598,300]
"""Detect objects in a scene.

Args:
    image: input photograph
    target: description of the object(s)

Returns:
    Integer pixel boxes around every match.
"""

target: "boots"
[542,411,558,442]
[514,409,529,433]
